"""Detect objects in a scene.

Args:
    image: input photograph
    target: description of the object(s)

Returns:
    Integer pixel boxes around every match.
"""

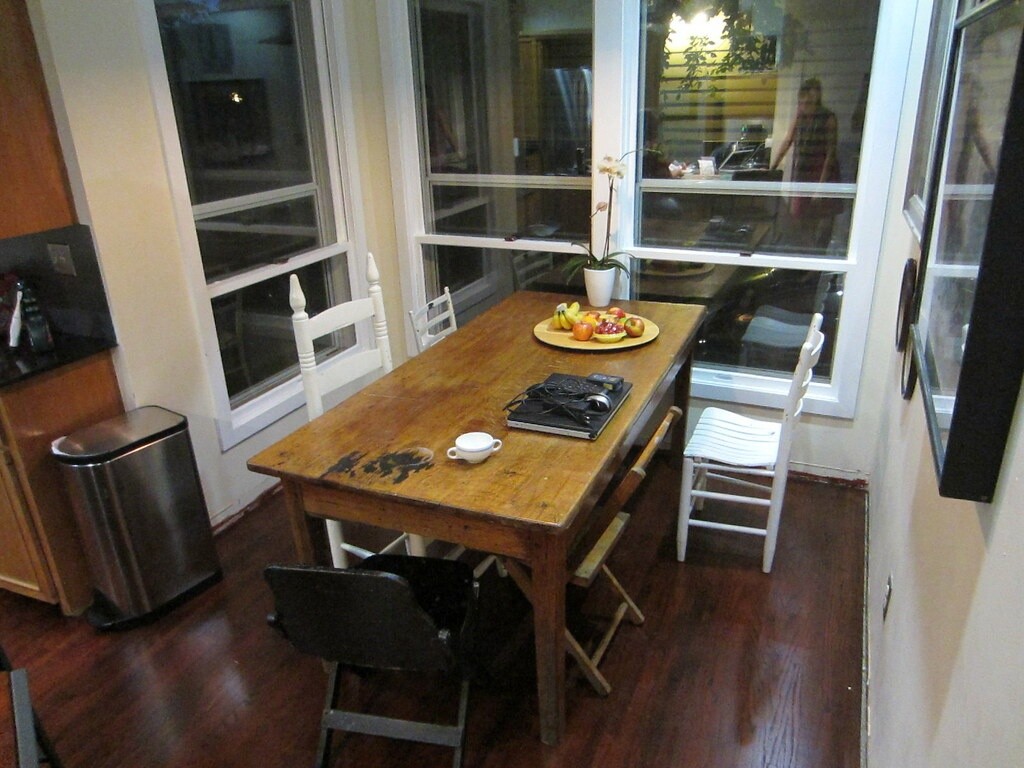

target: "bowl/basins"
[594,330,627,342]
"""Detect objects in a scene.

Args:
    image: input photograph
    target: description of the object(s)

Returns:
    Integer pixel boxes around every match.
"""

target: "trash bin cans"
[48,405,223,633]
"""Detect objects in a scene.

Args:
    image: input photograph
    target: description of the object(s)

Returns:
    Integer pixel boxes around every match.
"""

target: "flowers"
[555,139,666,284]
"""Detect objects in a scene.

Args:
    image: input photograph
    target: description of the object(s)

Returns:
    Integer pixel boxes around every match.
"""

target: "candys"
[595,319,624,334]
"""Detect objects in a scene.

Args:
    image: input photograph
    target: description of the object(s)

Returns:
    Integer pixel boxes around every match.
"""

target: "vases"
[582,263,618,309]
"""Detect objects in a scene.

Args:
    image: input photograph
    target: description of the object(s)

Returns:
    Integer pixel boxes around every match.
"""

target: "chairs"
[489,404,682,698]
[288,252,508,579]
[408,285,458,356]
[736,235,840,371]
[675,312,826,573]
[503,245,553,292]
[258,552,509,768]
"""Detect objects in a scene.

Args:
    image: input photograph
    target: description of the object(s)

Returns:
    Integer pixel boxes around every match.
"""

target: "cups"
[448,432,502,463]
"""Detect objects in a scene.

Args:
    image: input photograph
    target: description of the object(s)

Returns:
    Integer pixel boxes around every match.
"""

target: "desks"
[638,210,776,251]
[247,289,706,748]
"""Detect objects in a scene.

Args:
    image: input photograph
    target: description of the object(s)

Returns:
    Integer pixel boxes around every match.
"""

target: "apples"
[606,307,625,318]
[586,311,601,321]
[573,320,593,341]
[624,317,644,337]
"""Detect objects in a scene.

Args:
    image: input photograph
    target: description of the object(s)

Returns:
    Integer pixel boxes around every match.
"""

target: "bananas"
[552,302,585,329]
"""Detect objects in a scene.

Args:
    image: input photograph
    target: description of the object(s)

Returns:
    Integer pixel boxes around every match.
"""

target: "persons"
[712,121,764,169]
[949,106,997,263]
[643,130,687,217]
[760,79,843,286]
